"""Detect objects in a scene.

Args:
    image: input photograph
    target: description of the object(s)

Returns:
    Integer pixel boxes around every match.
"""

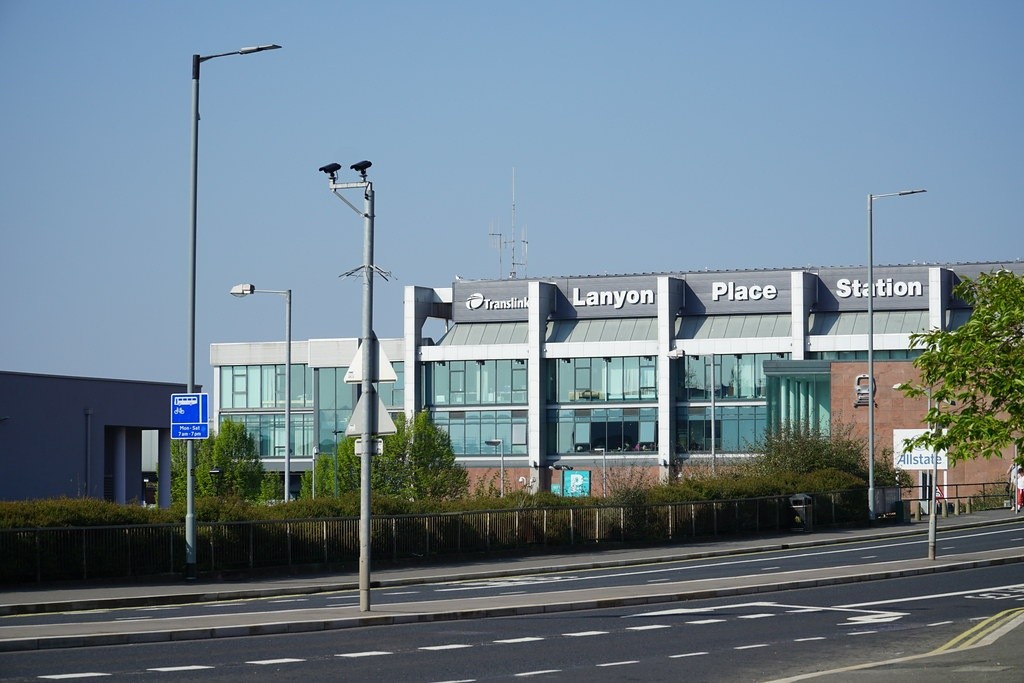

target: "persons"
[624,442,654,451]
[1007,457,1022,511]
[1014,468,1024,512]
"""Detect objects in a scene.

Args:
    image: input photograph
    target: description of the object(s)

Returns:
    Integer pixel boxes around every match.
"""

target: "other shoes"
[1011,508,1015,511]
[1014,510,1020,512]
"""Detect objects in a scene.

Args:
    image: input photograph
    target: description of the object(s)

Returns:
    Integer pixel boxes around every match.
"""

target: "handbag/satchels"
[1005,483,1009,493]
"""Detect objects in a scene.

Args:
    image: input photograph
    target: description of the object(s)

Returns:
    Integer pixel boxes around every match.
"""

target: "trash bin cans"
[788,493,813,534]
[875,486,901,520]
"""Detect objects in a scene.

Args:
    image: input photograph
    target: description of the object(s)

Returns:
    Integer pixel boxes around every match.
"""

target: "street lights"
[210,466,224,499]
[868,189,928,527]
[893,384,931,498]
[484,439,504,496]
[185,44,282,581]
[594,447,605,496]
[333,430,346,501]
[228,284,292,502]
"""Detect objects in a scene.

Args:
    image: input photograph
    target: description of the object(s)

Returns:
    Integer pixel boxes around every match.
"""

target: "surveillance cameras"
[350,161,372,171]
[319,163,342,173]
